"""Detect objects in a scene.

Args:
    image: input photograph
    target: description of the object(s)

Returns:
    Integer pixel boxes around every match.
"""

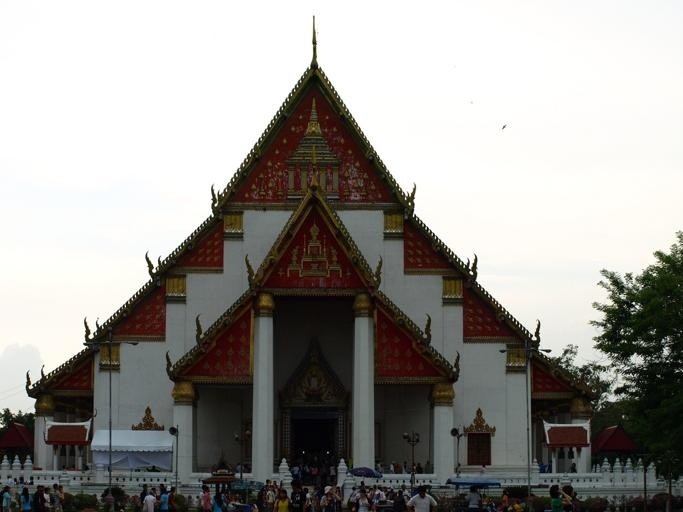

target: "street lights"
[498,335,552,497]
[402,430,419,493]
[82,327,140,512]
